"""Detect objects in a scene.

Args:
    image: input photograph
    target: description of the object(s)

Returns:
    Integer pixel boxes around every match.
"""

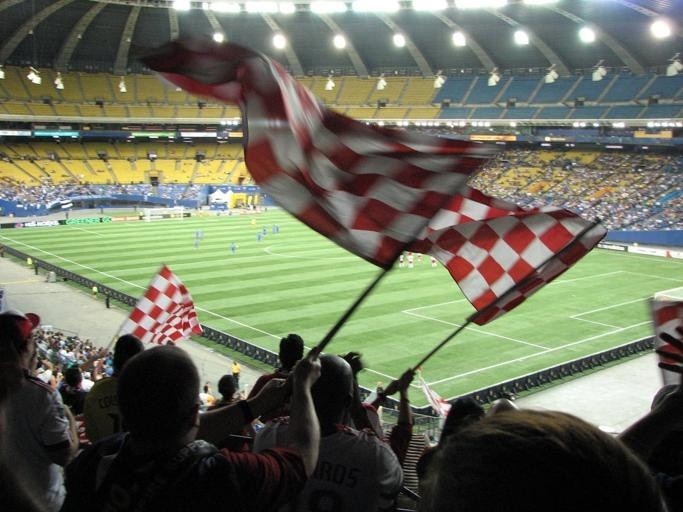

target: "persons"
[247,335,304,399]
[92,284,98,299]
[363,368,413,463]
[233,362,241,387]
[432,410,668,511]
[253,354,403,511]
[617,324,682,511]
[1,306,115,512]
[105,294,110,308]
[83,334,179,443]
[399,252,437,269]
[416,399,486,511]
[199,385,214,405]
[382,416,431,512]
[65,345,320,512]
[209,374,237,411]
[194,216,280,255]
[34,260,38,274]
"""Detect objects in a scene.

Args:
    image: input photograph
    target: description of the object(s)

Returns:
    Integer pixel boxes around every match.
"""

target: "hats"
[0,312,41,346]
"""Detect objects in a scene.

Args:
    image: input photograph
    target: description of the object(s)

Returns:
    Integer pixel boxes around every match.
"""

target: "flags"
[402,183,608,327]
[116,263,203,363]
[131,35,489,272]
[649,297,683,385]
